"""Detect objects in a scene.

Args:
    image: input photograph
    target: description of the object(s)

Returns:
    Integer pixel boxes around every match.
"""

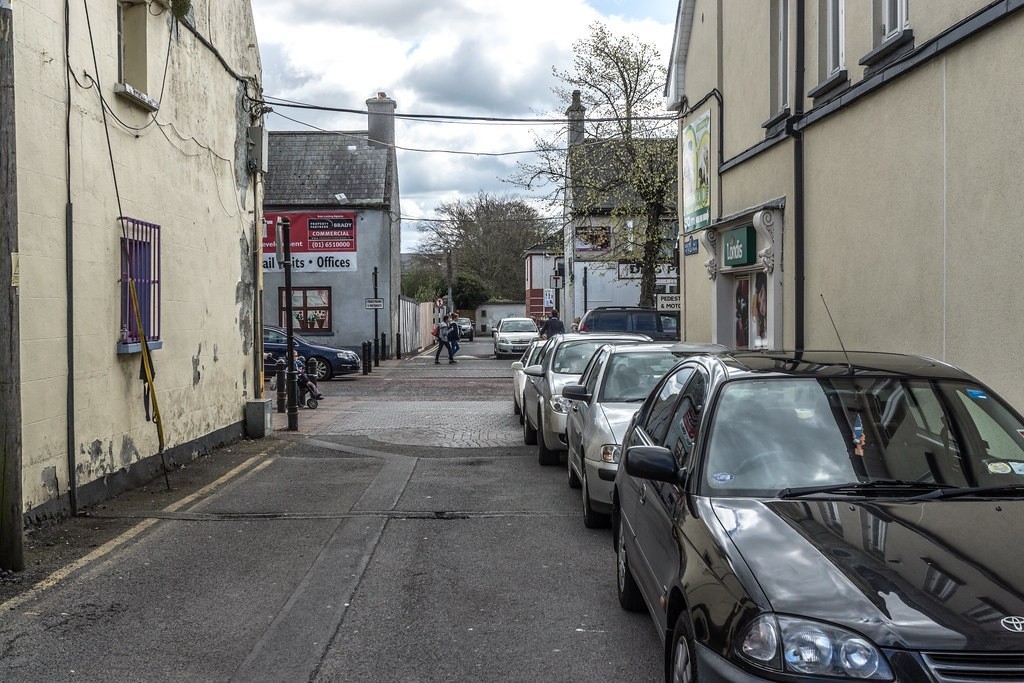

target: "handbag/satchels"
[269,374,277,390]
[430,325,440,337]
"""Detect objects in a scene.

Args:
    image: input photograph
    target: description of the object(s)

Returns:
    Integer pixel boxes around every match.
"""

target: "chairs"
[615,367,650,396]
[559,350,582,371]
[736,407,819,472]
[859,401,944,483]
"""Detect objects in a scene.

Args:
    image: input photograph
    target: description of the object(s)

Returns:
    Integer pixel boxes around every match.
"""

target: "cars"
[511,340,550,425]
[263,325,361,382]
[558,341,748,528]
[611,350,1024,683]
[491,318,541,359]
[521,332,655,466]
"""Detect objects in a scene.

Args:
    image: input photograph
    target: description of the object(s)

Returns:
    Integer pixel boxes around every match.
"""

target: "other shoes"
[449,361,457,364]
[448,356,454,360]
[434,361,441,365]
[315,394,324,400]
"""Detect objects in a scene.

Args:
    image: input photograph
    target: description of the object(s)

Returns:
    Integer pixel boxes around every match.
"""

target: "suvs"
[575,306,680,341]
[457,317,476,342]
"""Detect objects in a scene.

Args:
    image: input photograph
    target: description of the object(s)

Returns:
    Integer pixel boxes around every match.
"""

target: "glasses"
[293,355,298,357]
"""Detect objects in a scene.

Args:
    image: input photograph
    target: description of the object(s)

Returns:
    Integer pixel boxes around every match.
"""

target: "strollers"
[297,372,320,409]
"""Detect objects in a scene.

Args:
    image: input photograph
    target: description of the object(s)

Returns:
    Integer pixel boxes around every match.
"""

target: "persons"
[286,349,324,400]
[434,313,460,364]
[571,317,580,333]
[539,309,565,341]
